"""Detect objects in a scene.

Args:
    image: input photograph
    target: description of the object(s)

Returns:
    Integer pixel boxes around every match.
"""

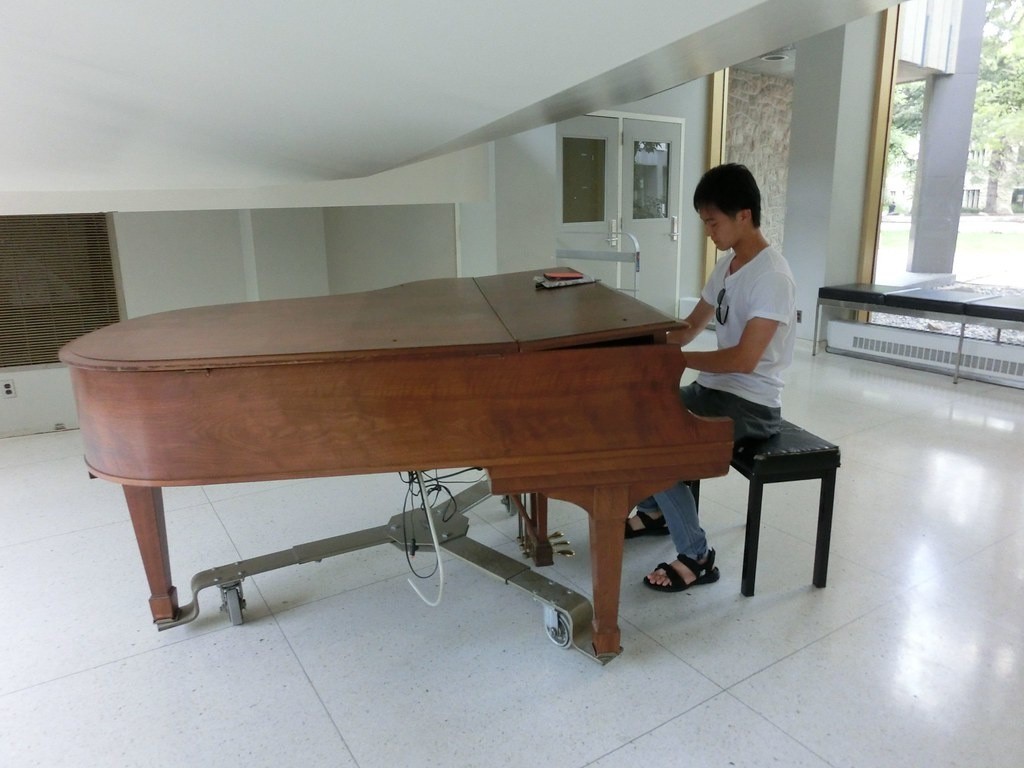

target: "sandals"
[644,547,720,591]
[625,511,670,539]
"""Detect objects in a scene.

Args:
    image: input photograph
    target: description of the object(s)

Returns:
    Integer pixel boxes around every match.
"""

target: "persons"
[624,165,796,593]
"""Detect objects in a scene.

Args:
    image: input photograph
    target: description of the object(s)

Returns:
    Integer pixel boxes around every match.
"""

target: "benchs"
[690,418,842,598]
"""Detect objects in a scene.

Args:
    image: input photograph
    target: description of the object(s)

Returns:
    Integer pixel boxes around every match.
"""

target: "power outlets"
[0,378,17,399]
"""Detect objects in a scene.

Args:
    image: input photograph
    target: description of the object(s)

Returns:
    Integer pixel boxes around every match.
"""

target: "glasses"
[716,288,729,325]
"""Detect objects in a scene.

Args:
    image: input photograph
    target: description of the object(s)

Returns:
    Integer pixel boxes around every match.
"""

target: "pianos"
[59,266,732,663]
